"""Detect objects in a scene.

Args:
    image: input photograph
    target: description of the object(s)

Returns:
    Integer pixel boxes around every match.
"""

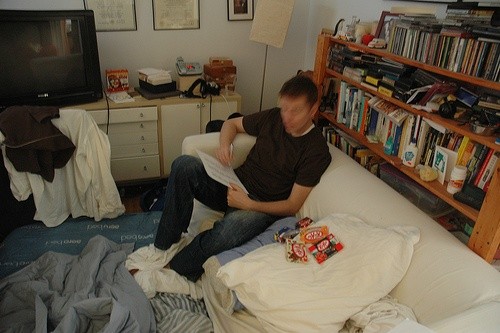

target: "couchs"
[182,133,500,333]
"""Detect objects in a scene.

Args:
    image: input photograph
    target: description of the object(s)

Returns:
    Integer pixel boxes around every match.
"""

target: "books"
[135,67,181,100]
[176,75,202,97]
[317,0,500,237]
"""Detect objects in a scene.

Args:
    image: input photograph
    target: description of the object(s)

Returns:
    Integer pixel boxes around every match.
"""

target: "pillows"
[215,212,421,333]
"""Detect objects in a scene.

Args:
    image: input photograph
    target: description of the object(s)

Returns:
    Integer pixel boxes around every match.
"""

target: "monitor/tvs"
[0,9,103,109]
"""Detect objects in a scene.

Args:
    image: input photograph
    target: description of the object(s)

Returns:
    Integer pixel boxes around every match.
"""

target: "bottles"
[447,165,467,194]
[384,136,394,154]
[402,138,418,167]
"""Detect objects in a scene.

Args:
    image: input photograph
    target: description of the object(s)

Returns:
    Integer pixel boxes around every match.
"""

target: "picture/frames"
[227,0,254,21]
[374,11,406,42]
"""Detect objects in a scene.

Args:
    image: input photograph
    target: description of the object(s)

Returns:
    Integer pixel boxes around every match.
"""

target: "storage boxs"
[139,78,176,93]
[176,75,201,92]
[379,163,453,218]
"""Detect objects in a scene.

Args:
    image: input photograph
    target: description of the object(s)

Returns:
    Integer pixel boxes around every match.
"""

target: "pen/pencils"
[227,142,236,166]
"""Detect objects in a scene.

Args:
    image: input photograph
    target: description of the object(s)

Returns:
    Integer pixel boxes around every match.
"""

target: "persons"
[153,76,332,282]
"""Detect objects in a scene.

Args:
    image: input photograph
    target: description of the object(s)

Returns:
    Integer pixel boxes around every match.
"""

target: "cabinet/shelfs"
[60,87,241,187]
[313,35,500,264]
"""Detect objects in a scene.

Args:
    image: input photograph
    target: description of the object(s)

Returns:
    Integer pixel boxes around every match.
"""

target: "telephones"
[174,54,204,76]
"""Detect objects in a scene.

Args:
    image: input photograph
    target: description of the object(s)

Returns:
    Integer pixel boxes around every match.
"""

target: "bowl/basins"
[470,122,496,136]
